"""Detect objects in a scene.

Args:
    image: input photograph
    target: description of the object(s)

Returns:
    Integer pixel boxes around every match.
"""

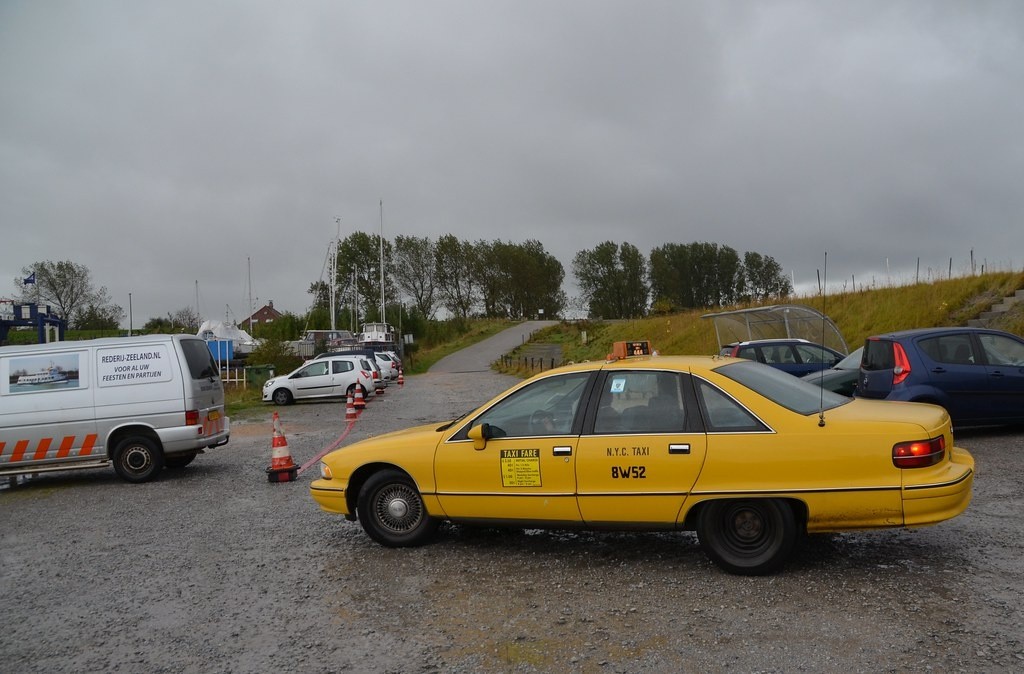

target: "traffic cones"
[345,388,358,421]
[267,410,301,482]
[352,377,366,409]
[376,388,384,394]
[397,366,405,385]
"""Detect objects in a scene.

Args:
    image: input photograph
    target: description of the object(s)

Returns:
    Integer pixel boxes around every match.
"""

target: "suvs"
[261,357,375,407]
[299,350,403,388]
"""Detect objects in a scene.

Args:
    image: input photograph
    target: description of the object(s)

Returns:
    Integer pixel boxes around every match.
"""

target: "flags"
[24,272,35,285]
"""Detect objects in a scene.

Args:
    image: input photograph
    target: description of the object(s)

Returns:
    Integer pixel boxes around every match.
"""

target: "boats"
[17,367,69,384]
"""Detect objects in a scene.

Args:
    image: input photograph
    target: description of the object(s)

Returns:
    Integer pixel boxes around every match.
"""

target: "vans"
[0,334,230,485]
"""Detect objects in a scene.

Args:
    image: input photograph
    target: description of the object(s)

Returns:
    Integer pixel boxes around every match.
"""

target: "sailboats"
[299,200,397,342]
[193,255,263,353]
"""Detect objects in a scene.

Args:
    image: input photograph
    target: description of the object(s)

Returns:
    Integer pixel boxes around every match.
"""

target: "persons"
[543,384,615,431]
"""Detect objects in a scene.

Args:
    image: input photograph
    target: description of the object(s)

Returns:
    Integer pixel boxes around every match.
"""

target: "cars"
[308,339,976,577]
[802,345,864,398]
[719,340,846,378]
[856,327,1024,432]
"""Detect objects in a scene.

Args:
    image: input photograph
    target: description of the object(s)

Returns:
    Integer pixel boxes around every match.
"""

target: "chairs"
[592,386,617,431]
[953,344,971,365]
